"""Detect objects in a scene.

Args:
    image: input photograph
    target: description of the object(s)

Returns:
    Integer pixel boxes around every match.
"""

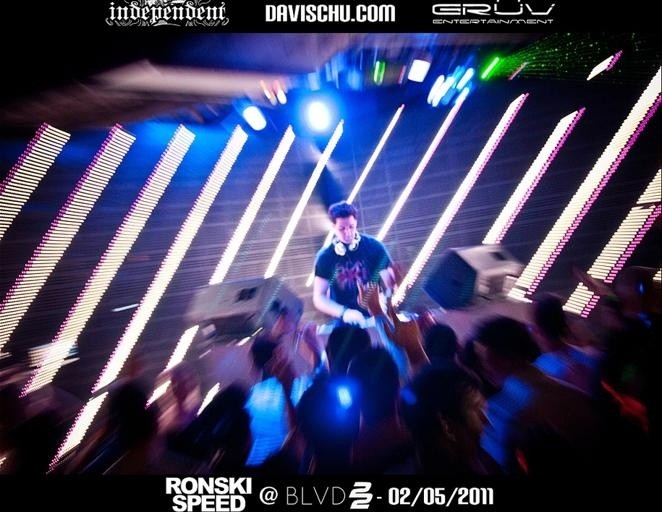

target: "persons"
[309,201,399,329]
[0,263,662,511]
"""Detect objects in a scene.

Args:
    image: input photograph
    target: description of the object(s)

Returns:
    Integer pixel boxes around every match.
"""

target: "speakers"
[421,242,524,311]
[182,276,306,342]
